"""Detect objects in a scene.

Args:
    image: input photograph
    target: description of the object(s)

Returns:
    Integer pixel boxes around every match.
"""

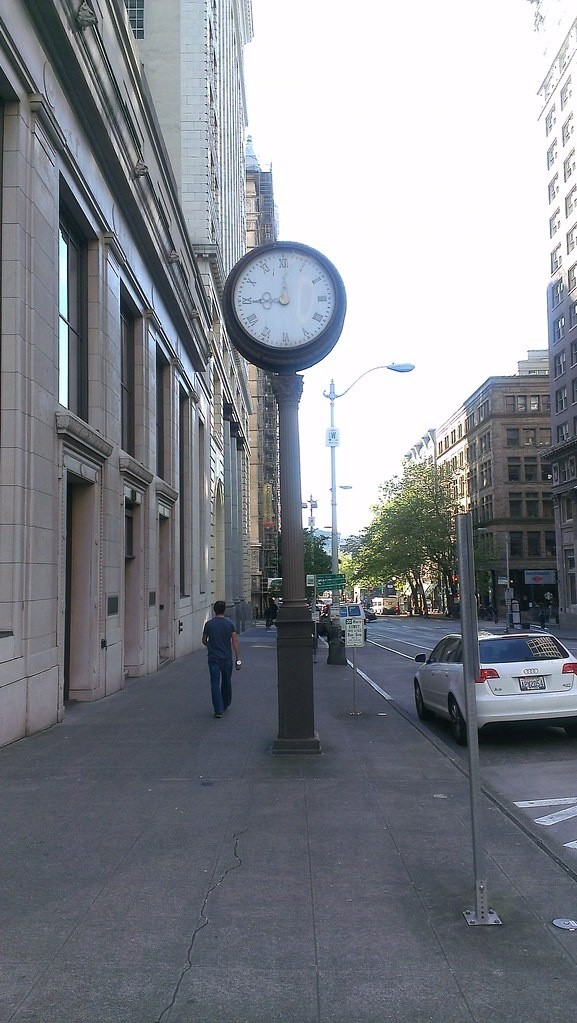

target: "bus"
[371,597,398,614]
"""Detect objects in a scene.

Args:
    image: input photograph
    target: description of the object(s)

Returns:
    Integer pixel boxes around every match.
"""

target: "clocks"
[222,240,346,361]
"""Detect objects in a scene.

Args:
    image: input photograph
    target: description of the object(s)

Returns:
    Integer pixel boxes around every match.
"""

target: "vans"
[321,603,367,641]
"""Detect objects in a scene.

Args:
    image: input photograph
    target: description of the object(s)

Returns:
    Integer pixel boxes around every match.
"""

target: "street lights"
[477,528,515,629]
[307,485,354,613]
[325,362,417,665]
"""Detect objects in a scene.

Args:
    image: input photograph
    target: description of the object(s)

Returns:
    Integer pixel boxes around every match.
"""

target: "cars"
[308,604,327,637]
[413,628,577,745]
[363,609,378,623]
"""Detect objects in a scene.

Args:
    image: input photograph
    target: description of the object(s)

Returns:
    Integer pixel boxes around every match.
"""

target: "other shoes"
[214,712,222,717]
[224,706,229,712]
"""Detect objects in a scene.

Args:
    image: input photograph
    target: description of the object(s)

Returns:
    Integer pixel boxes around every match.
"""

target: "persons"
[202,600,243,719]
[264,606,272,630]
[538,611,545,630]
[479,602,499,624]
[268,599,278,626]
